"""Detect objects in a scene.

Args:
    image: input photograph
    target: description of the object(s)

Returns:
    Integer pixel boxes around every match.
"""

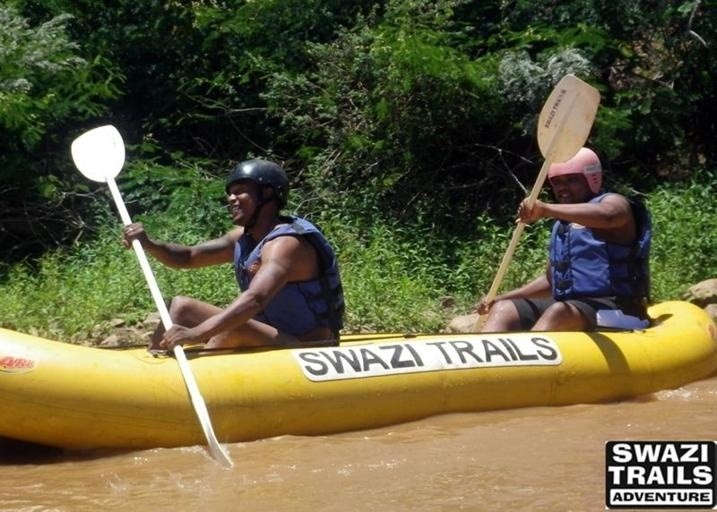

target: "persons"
[481,147,653,334]
[122,159,345,352]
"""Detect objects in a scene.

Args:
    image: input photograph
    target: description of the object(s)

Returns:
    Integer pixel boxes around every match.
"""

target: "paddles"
[69,123,234,468]
[472,73,602,332]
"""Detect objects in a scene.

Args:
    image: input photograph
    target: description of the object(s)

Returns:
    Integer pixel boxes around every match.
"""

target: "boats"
[0,301,717,448]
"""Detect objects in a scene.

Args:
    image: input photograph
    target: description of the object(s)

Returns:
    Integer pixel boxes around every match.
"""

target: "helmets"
[223,158,291,209]
[547,147,603,194]
